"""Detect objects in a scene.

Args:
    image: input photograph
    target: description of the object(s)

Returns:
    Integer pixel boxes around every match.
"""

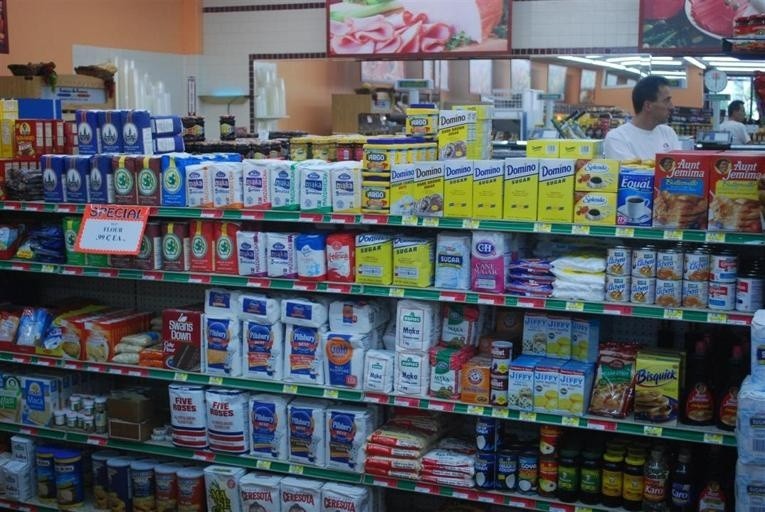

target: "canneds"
[182,114,367,160]
[490,342,512,406]
[605,244,763,313]
[475,418,647,512]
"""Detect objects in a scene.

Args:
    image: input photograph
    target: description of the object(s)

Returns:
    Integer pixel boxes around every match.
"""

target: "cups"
[625,195,650,218]
[104,54,174,118]
[586,209,603,222]
[588,176,606,190]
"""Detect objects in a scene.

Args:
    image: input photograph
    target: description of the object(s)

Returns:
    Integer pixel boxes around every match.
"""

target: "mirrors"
[248,46,765,163]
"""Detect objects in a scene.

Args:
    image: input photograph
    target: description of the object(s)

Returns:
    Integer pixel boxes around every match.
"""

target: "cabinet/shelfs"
[0,200,765,512]
[331,92,395,135]
[0,75,116,114]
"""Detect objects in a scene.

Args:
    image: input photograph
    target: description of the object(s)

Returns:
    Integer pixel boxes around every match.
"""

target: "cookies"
[508,388,534,409]
[524,333,547,354]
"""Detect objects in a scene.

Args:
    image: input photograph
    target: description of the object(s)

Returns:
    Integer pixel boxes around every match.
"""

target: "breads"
[547,337,570,355]
[571,340,590,360]
[535,391,558,410]
[559,393,583,414]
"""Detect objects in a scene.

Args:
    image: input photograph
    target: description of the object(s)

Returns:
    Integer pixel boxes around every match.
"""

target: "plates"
[618,204,652,225]
[684,1,765,40]
[330,0,482,54]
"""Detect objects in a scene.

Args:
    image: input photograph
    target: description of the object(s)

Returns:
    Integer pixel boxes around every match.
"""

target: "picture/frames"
[637,0,765,52]
[0,0,9,54]
[325,0,512,58]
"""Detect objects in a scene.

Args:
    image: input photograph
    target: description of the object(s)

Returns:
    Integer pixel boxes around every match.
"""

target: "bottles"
[642,441,697,512]
[685,334,742,433]
[50,390,108,432]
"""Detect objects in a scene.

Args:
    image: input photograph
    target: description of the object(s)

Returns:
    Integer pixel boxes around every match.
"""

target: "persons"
[718,100,752,146]
[604,75,681,161]
[599,112,611,136]
